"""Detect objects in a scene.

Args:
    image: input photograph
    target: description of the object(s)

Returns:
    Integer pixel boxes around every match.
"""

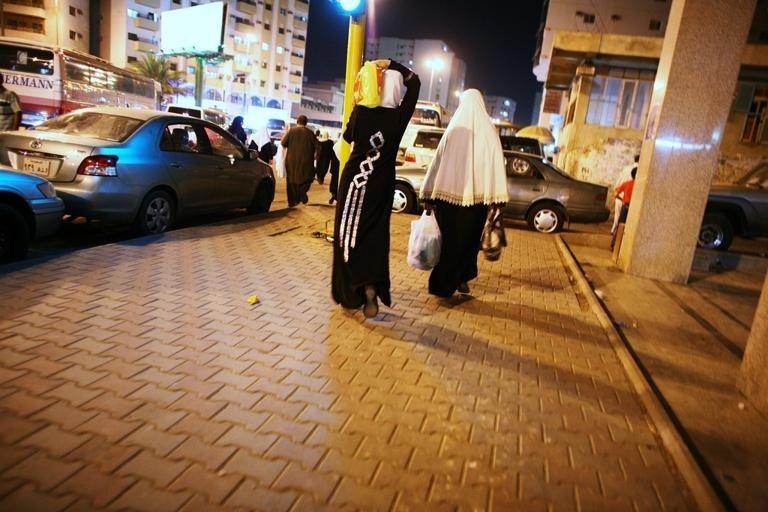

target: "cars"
[697,157,767,251]
[391,99,610,234]
[0,163,65,264]
[0,105,275,241]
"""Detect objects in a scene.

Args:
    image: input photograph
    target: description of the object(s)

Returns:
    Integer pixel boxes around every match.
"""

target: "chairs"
[172,129,189,152]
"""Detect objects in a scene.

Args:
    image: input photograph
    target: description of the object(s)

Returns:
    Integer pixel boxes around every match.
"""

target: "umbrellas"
[514,126,556,145]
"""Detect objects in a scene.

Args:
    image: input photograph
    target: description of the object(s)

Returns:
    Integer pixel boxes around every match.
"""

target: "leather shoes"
[363,286,378,318]
[458,281,470,293]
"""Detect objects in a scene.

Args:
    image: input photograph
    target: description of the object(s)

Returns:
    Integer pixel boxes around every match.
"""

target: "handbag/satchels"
[480,204,507,262]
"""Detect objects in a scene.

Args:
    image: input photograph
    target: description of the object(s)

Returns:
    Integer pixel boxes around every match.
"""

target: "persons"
[405,88,510,299]
[331,58,421,321]
[226,115,341,207]
[609,166,638,252]
[0,73,23,166]
[609,155,640,235]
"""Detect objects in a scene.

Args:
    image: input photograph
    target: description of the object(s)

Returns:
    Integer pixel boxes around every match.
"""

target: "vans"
[165,103,225,144]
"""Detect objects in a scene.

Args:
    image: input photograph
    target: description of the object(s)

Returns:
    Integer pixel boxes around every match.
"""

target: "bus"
[0,35,162,131]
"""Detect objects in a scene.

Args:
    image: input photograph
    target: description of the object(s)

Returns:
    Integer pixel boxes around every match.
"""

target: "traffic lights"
[335,0,365,16]
